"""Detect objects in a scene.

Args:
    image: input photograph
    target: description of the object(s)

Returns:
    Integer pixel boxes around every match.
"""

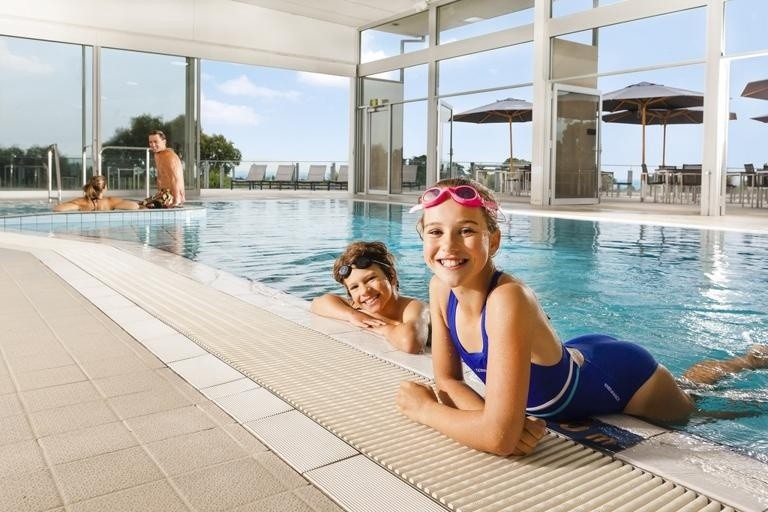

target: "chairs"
[474,163,531,199]
[402,164,423,191]
[231,165,267,190]
[295,164,326,191]
[264,165,295,189]
[726,163,768,209]
[327,165,348,192]
[600,163,702,208]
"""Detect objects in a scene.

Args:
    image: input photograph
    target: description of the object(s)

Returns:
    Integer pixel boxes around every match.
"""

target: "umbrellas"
[447,96,534,170]
[599,80,734,165]
[738,78,768,101]
[748,114,768,124]
[601,105,738,166]
[556,92,601,198]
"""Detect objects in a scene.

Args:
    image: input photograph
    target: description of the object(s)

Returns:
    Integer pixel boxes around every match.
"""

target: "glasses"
[417,184,500,209]
[338,256,391,278]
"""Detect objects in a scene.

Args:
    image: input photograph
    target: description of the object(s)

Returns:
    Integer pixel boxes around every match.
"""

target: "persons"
[138,129,187,209]
[51,175,139,214]
[308,240,431,359]
[394,176,768,460]
[153,223,186,255]
